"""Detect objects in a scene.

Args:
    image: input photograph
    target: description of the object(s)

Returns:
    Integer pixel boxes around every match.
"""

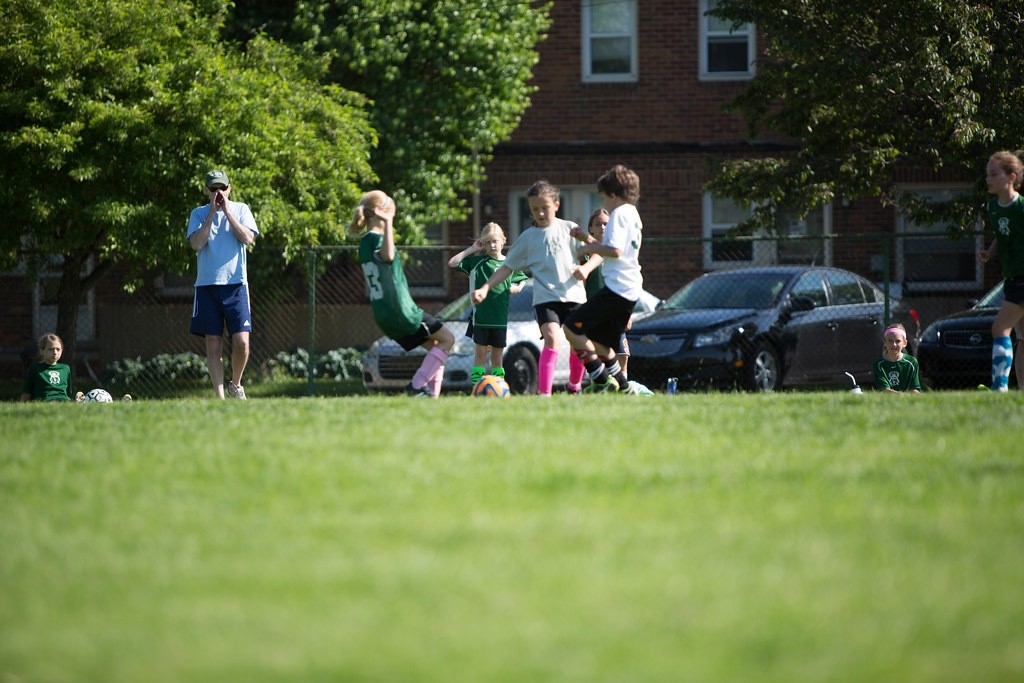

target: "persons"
[185,170,258,399]
[19,334,73,403]
[349,189,454,398]
[471,180,616,395]
[568,208,631,395]
[872,322,921,393]
[562,164,643,394]
[448,223,527,388]
[978,150,1024,394]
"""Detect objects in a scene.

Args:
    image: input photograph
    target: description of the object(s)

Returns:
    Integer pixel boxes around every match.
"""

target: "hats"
[205,170,229,187]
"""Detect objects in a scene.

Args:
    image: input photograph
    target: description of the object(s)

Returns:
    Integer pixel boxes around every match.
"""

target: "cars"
[917,277,1016,388]
[363,265,672,398]
[623,263,922,395]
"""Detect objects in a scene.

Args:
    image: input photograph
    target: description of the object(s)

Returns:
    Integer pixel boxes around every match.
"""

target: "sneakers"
[403,382,433,398]
[582,375,620,395]
[619,383,635,395]
[226,381,246,400]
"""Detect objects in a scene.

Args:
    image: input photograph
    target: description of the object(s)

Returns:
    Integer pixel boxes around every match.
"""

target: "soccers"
[84,388,114,404]
[471,374,511,400]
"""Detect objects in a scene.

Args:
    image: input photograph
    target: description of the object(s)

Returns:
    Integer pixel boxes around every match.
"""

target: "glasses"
[205,183,229,192]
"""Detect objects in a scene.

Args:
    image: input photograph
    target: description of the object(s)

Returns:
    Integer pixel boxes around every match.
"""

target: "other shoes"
[978,384,994,391]
[123,394,133,402]
[75,392,85,402]
